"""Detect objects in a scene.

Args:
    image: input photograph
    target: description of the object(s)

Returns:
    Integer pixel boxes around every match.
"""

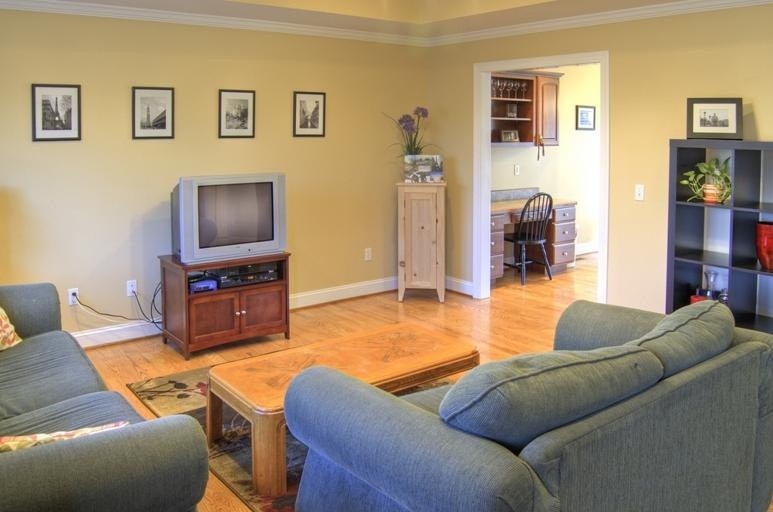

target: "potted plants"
[677,152,730,205]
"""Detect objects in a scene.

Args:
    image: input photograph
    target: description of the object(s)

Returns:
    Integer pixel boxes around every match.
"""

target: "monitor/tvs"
[170,173,286,267]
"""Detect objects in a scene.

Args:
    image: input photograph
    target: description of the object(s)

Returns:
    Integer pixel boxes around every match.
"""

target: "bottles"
[690,269,728,306]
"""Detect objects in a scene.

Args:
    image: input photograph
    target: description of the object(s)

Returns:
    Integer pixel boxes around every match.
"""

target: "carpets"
[122,358,455,511]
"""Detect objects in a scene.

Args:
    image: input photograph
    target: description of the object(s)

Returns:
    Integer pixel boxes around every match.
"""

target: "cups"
[505,103,519,118]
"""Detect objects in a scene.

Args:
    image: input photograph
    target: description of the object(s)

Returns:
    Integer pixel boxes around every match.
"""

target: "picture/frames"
[574,105,596,132]
[130,84,175,142]
[291,90,326,138]
[216,88,255,139]
[30,80,81,142]
[684,96,744,141]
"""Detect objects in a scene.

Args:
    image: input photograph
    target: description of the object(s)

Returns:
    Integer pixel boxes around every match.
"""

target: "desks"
[489,186,579,283]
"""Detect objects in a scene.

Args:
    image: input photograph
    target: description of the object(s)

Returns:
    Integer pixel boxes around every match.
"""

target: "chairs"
[503,192,561,287]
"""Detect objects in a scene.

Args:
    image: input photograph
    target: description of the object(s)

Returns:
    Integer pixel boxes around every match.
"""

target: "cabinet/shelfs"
[491,71,537,146]
[532,67,566,147]
[154,251,292,362]
[393,179,450,304]
[662,137,773,331]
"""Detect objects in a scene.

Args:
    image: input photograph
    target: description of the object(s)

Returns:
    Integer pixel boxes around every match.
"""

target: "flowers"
[380,103,443,158]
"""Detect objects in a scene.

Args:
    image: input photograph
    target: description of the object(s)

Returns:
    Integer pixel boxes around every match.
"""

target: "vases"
[753,220,773,273]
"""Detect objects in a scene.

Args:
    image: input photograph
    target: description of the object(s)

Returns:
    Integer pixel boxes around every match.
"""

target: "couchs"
[0,276,211,512]
[282,299,773,511]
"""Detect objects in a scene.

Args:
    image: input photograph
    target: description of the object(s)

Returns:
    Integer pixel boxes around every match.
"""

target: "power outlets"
[125,279,136,299]
[67,288,78,306]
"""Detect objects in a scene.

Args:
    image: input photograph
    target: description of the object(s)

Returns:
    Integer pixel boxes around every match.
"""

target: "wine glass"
[490,78,529,99]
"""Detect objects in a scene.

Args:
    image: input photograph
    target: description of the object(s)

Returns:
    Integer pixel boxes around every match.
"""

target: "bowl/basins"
[755,221,773,272]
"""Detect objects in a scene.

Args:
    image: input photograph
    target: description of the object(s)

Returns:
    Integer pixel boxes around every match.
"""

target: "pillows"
[0,418,128,453]
[0,306,24,351]
